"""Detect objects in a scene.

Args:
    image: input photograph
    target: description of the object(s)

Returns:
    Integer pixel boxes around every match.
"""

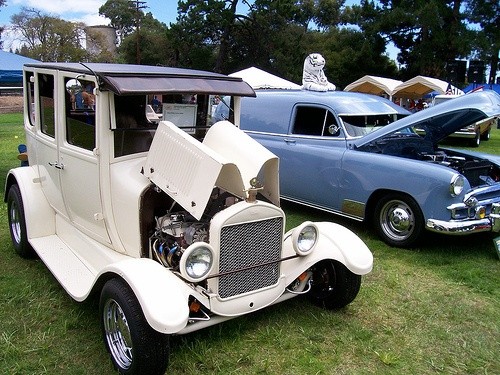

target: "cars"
[5,59,375,375]
[213,52,500,246]
